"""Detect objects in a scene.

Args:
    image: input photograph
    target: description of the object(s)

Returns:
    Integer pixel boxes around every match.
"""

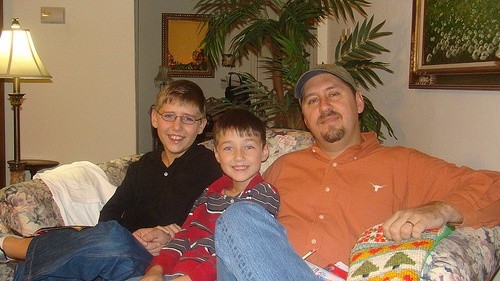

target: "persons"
[215,63,500,281]
[0,79,228,281]
[139,108,280,281]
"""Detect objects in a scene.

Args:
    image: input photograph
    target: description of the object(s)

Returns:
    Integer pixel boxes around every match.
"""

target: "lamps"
[0,17,54,183]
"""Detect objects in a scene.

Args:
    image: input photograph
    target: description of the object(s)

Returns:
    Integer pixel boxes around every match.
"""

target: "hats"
[293,64,357,98]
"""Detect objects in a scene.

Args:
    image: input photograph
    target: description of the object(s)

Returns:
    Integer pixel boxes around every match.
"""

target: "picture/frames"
[161,12,215,78]
[408,0,499,91]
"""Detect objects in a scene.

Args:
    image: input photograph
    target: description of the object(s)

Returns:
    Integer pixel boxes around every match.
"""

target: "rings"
[406,221,414,226]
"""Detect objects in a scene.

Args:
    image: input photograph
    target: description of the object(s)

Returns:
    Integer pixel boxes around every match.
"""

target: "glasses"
[158,111,202,125]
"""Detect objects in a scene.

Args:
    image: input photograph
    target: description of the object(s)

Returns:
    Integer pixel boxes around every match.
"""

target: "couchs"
[0,129,500,281]
